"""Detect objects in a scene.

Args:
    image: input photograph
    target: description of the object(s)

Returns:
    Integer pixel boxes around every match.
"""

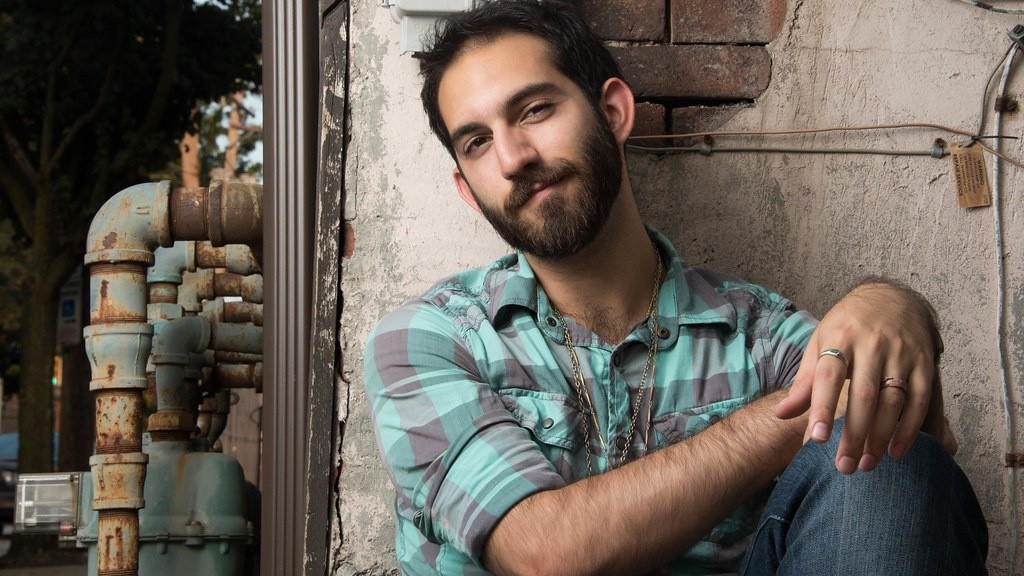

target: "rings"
[818,349,850,369]
[879,375,909,394]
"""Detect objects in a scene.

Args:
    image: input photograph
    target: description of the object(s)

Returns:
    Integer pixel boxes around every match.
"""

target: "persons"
[363,0,993,576]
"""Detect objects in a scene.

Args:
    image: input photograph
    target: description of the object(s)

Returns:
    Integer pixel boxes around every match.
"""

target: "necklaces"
[550,239,663,479]
